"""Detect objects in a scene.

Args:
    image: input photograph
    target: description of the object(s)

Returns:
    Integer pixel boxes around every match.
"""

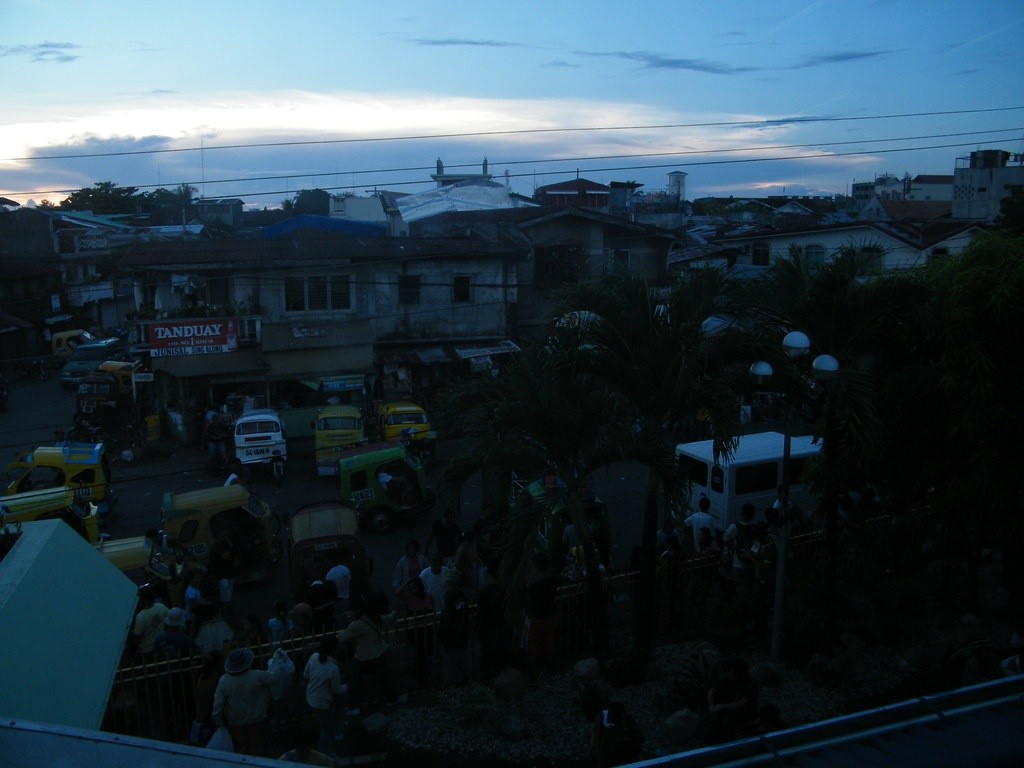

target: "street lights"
[747,333,841,677]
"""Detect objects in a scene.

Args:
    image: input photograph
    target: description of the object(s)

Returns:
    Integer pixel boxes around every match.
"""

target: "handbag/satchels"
[206,727,234,752]
[190,719,209,746]
[268,648,295,680]
[272,678,294,713]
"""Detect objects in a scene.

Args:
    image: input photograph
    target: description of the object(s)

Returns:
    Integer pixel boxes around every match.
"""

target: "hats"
[224,648,253,673]
[445,570,463,583]
[165,607,186,626]
[54,428,64,434]
[396,427,414,440]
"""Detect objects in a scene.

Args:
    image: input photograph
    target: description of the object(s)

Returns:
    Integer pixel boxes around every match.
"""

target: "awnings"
[256,342,384,379]
[377,341,518,362]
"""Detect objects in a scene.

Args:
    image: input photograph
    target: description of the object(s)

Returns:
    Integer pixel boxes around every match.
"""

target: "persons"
[125,309,135,321]
[0,358,1024,768]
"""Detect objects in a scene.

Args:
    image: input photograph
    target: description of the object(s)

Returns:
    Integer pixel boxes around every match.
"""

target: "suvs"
[56,336,128,388]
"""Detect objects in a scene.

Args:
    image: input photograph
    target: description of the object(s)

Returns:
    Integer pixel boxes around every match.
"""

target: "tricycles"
[280,500,372,608]
[0,482,99,553]
[1,313,98,387]
[334,447,434,536]
[161,483,284,580]
[67,360,145,447]
[3,439,113,506]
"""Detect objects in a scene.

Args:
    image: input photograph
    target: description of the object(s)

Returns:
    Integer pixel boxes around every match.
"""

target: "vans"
[671,429,827,542]
[231,409,288,469]
[310,405,369,466]
[373,401,437,449]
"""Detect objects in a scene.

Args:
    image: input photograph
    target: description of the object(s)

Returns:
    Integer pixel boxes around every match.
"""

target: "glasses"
[343,616,353,620]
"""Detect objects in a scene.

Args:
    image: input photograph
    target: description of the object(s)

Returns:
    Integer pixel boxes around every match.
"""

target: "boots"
[208,463,215,476]
[219,459,225,470]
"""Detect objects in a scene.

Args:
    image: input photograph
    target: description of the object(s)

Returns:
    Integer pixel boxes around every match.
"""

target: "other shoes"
[385,698,400,706]
[334,733,343,741]
[345,704,361,716]
